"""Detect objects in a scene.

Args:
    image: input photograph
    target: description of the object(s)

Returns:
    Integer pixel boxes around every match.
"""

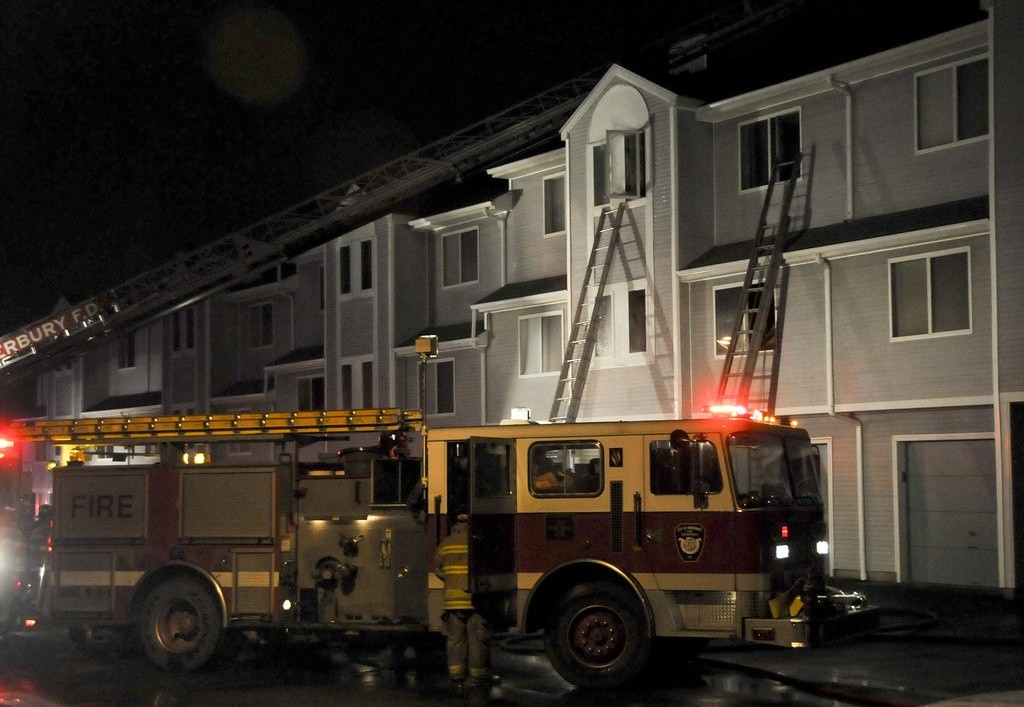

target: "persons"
[432,515,491,697]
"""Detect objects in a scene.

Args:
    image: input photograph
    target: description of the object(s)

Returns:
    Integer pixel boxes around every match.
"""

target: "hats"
[456,513,469,523]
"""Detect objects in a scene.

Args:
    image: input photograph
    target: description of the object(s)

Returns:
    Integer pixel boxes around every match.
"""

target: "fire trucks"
[0,0,883,691]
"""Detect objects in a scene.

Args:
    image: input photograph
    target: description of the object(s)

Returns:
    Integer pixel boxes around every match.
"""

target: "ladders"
[548,203,627,423]
[716,150,803,410]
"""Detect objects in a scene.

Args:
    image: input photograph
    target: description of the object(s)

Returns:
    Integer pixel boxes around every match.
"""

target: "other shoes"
[473,674,501,688]
[451,679,464,688]
